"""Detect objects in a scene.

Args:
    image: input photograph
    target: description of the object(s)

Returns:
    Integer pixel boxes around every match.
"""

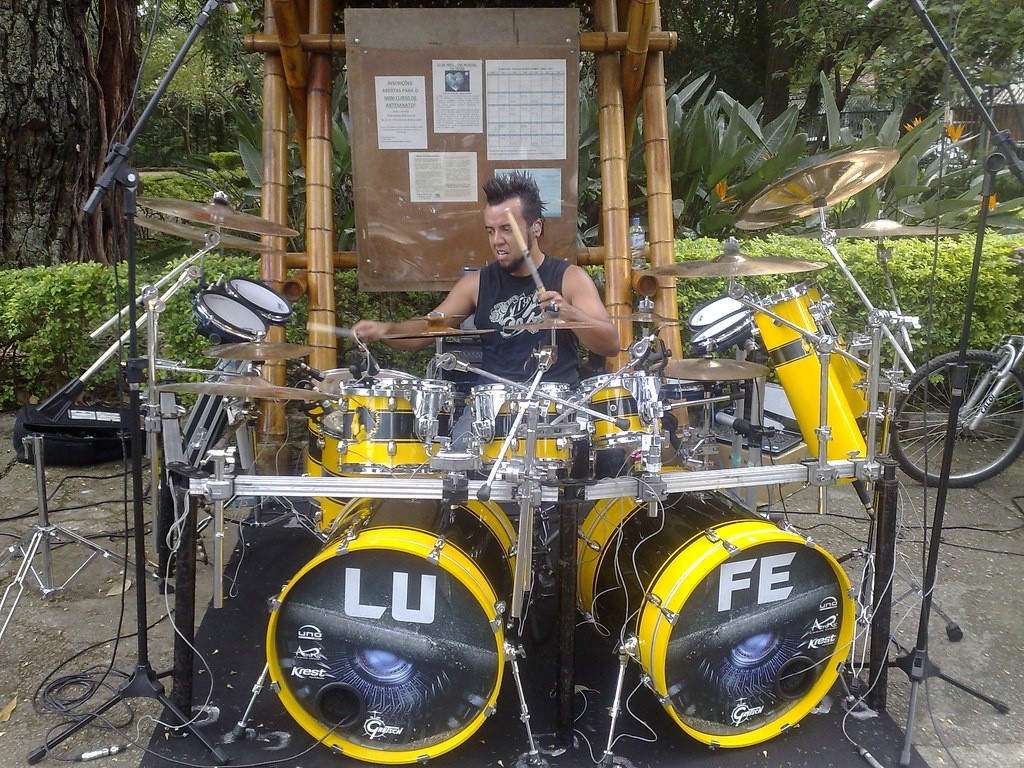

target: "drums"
[689,290,766,354]
[265,491,528,765]
[749,281,870,488]
[217,276,294,328]
[569,371,666,454]
[192,291,269,346]
[299,365,454,542]
[468,382,580,472]
[572,466,858,749]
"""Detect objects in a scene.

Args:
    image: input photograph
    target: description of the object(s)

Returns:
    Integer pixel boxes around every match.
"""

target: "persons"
[350,170,620,454]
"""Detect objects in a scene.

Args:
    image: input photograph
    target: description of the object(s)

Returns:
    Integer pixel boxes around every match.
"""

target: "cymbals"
[375,313,499,345]
[633,251,831,279]
[126,207,296,256]
[609,310,686,336]
[128,189,301,241]
[813,215,960,242]
[648,346,772,384]
[501,317,606,339]
[732,145,903,232]
[194,340,314,363]
[152,369,334,408]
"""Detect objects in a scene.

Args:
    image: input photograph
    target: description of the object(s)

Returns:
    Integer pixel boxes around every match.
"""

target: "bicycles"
[890,333,1023,488]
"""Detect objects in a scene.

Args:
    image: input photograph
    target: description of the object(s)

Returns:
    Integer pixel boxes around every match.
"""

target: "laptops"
[714,381,804,456]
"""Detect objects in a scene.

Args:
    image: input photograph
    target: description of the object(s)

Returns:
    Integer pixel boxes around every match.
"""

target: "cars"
[806,136,862,150]
[919,135,977,172]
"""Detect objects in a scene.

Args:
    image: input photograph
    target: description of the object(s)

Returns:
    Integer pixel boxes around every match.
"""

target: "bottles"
[629,218,646,269]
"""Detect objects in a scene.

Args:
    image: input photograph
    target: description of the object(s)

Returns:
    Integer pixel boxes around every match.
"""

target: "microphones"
[355,334,380,376]
[634,328,660,371]
[867,0,886,12]
[855,743,884,768]
[716,412,754,434]
[297,360,326,382]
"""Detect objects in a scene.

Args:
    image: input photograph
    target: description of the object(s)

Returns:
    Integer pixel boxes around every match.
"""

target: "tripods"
[0,0,242,767]
[727,0,1024,767]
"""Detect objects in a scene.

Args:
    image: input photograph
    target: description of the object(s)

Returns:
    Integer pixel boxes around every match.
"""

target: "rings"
[550,300,555,307]
[555,305,557,311]
